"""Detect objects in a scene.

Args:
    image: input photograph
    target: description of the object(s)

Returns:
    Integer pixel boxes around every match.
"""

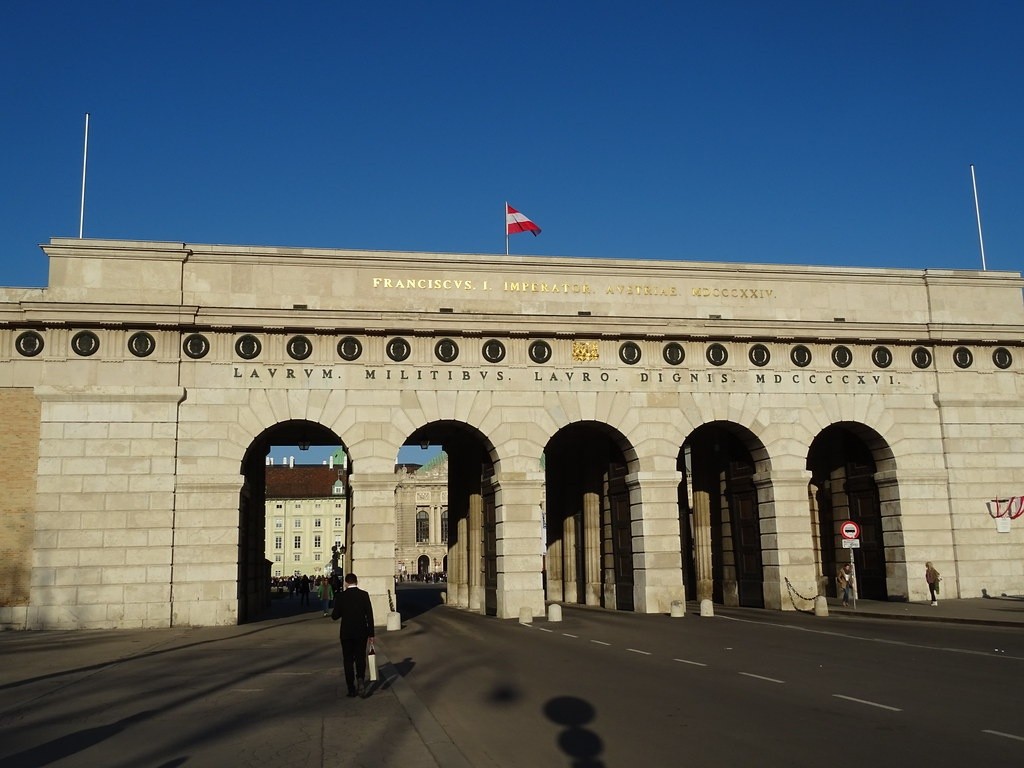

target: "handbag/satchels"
[368,642,379,680]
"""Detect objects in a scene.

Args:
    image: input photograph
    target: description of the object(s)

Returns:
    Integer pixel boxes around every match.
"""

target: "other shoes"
[346,691,356,697]
[357,678,365,697]
[324,613,328,616]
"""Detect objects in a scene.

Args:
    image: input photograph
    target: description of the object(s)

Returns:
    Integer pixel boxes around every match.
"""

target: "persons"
[400,572,447,584]
[271,575,343,606]
[508,205,542,237]
[331,573,375,698]
[317,578,334,617]
[837,563,854,607]
[925,561,941,606]
